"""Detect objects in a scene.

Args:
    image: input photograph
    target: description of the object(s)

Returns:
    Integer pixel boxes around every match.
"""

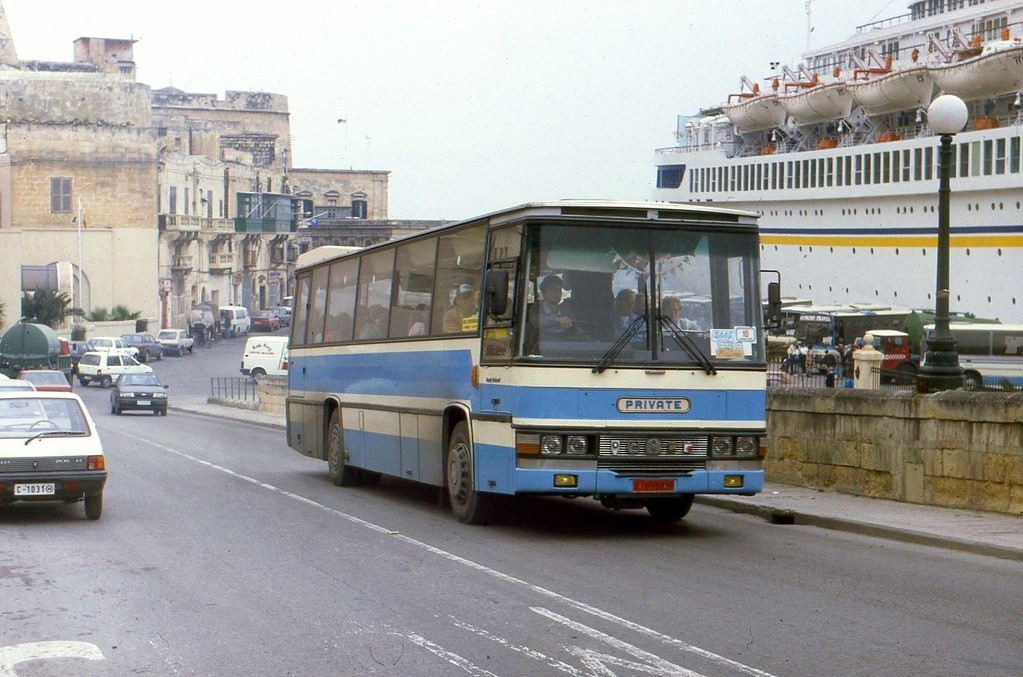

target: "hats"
[455,284,474,296]
[835,346,838,349]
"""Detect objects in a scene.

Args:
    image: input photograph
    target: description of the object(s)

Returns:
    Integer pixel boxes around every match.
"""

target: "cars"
[250,306,290,332]
[109,372,170,416]
[0,369,108,519]
[69,332,169,388]
[156,329,194,357]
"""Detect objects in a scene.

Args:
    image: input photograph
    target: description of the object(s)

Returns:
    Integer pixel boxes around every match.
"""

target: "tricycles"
[188,301,222,347]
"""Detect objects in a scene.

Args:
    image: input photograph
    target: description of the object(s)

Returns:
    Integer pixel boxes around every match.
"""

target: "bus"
[662,290,920,360]
[918,323,1023,389]
[284,198,780,522]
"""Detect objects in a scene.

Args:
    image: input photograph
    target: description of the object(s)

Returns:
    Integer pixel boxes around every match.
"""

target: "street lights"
[914,95,969,388]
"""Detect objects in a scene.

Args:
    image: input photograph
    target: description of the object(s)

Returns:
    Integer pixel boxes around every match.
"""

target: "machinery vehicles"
[851,329,916,384]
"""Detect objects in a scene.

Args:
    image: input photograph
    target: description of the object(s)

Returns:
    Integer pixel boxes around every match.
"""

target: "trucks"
[0,319,82,388]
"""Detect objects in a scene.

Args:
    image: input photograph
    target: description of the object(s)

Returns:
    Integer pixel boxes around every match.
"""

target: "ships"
[647,0,1023,324]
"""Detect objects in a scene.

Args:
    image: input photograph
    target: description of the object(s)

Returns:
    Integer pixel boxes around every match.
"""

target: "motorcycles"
[240,336,290,384]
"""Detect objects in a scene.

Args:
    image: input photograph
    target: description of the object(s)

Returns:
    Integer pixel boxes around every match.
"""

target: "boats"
[845,49,935,116]
[719,76,786,133]
[779,63,854,126]
[926,27,1023,102]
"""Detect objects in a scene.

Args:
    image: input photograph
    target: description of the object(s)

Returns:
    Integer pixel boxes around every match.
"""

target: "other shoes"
[806,374,810,377]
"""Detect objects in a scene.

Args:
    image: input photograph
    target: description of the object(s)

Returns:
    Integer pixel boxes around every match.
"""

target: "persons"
[560,270,616,342]
[407,303,427,336]
[820,343,860,389]
[528,274,597,341]
[612,289,647,342]
[660,297,704,338]
[442,283,476,334]
[633,292,653,317]
[785,339,814,376]
[296,303,384,343]
[222,314,231,338]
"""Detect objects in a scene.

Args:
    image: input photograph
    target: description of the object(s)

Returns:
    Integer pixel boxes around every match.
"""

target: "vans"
[219,306,251,338]
[277,296,293,313]
